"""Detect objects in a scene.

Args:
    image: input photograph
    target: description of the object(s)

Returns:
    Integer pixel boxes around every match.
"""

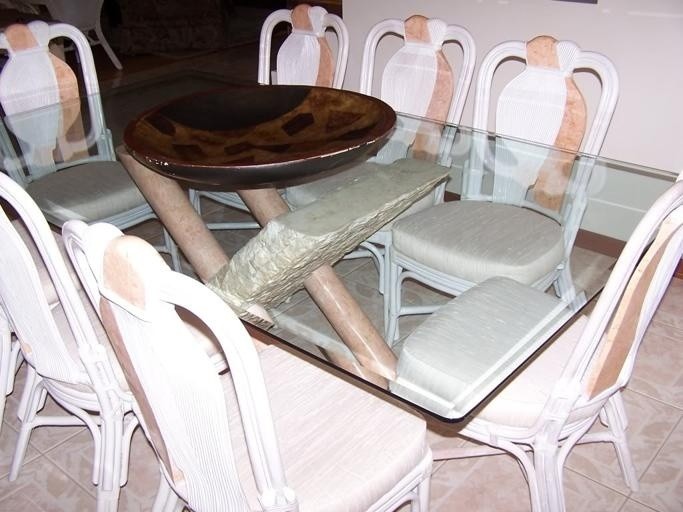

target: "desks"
[0,75,681,420]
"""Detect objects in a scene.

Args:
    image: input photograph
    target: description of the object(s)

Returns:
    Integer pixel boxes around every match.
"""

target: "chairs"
[1,218,84,396]
[0,20,181,270]
[278,14,477,295]
[385,175,682,512]
[190,5,350,228]
[45,1,123,70]
[61,219,434,512]
[385,35,620,302]
[0,173,266,512]
